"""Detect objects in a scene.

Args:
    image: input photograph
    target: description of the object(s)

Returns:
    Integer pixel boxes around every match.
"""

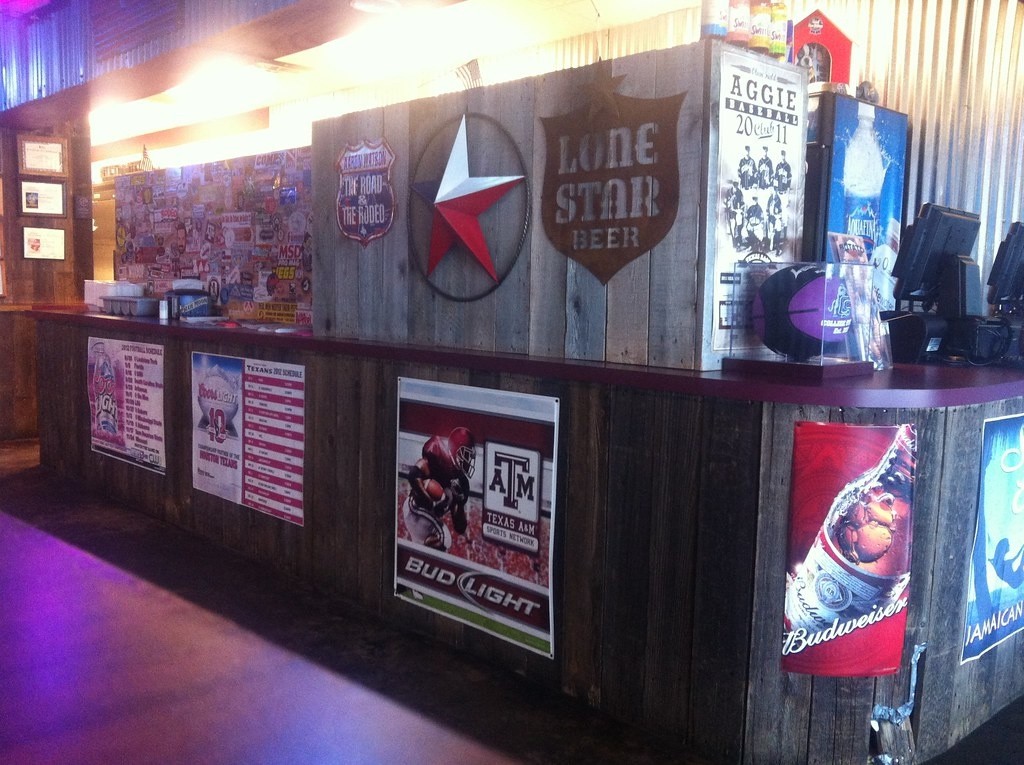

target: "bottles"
[726,0,750,49]
[782,425,917,636]
[700,0,728,43]
[843,102,884,263]
[749,0,771,55]
[159,300,169,320]
[770,0,788,62]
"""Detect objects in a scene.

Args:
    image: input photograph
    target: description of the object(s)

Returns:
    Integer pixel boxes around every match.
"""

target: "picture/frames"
[17,177,67,218]
[22,226,66,261]
[17,134,69,179]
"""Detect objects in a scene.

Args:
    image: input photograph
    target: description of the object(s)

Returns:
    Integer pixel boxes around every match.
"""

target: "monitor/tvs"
[893,201,981,316]
[986,222,1024,306]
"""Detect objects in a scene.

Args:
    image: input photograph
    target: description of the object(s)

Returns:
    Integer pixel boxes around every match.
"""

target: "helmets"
[448,427,474,472]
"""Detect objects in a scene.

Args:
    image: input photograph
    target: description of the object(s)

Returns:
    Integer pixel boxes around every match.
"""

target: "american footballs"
[751,263,852,360]
[423,478,447,502]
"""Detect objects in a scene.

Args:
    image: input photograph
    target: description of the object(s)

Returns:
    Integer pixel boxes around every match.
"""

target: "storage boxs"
[84,279,145,308]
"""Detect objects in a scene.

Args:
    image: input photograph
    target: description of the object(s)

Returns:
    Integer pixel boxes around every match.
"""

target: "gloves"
[433,494,448,516]
